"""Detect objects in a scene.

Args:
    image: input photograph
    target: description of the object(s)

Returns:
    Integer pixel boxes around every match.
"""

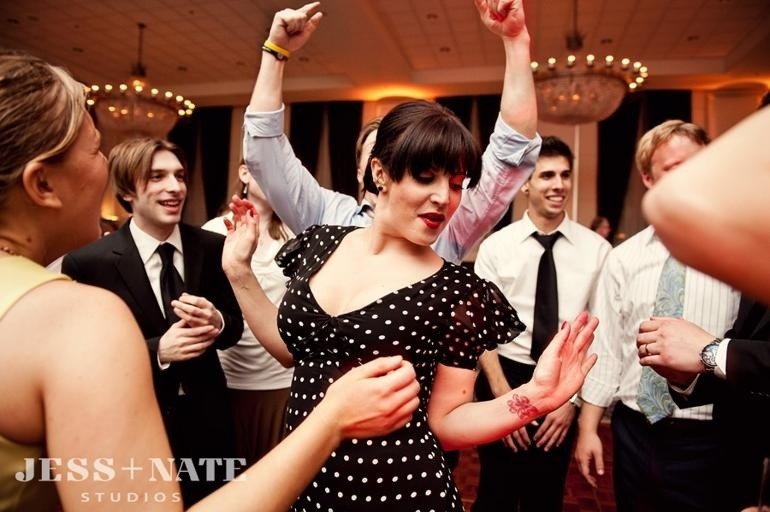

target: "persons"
[573,118,743,512]
[0,54,422,512]
[60,136,243,511]
[242,0,543,471]
[200,158,298,472]
[470,135,613,512]
[221,99,600,511]
[635,297,770,512]
[640,90,769,305]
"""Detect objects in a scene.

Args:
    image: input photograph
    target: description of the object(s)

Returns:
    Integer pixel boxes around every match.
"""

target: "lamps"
[523,1,649,127]
[86,23,197,138]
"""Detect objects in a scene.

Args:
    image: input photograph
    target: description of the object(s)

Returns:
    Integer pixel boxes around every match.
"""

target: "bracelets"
[261,41,290,60]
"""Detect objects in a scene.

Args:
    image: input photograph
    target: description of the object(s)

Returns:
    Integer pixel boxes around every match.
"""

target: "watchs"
[700,338,722,373]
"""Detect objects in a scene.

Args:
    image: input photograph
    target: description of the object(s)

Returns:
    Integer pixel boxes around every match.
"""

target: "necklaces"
[0,242,13,255]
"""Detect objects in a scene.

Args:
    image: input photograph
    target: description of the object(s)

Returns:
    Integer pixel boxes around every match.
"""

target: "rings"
[645,344,648,352]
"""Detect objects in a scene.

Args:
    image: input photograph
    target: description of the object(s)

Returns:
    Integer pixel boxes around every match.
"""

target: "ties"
[153,241,189,329]
[632,245,689,428]
[526,229,563,369]
